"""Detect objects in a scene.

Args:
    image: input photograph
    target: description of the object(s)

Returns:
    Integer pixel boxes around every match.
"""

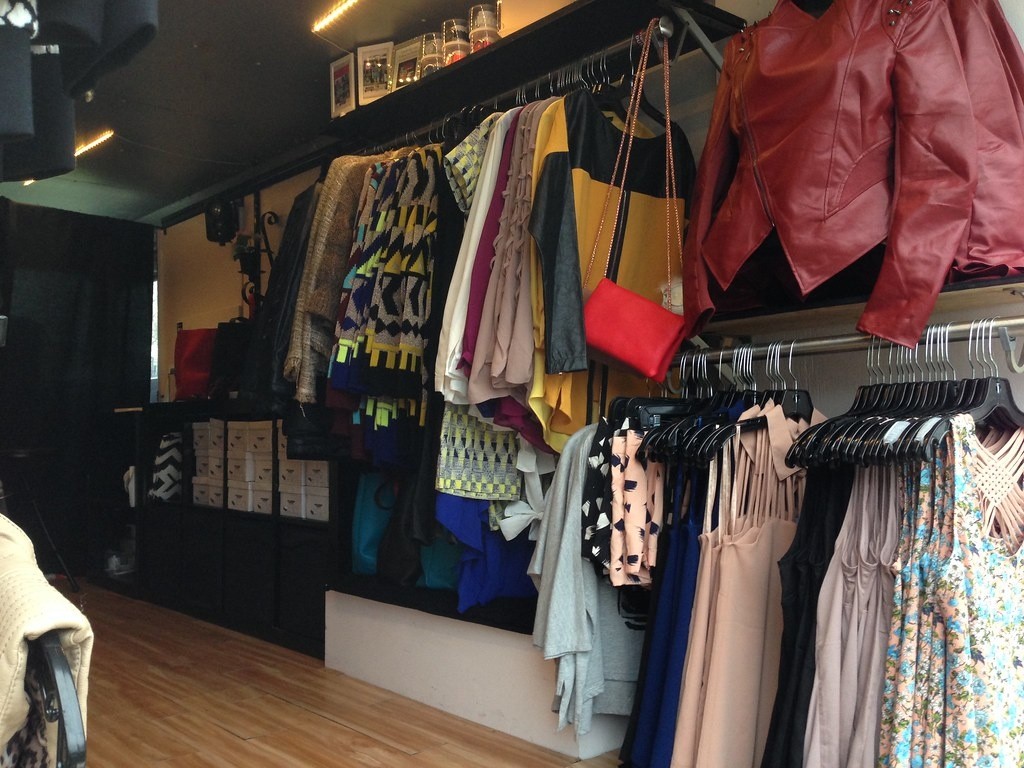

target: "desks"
[0,447,103,593]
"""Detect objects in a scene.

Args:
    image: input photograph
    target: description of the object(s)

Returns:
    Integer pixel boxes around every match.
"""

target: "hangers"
[294,0,1024,465]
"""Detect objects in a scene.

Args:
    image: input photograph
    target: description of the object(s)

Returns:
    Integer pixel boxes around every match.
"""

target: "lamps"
[311,0,359,33]
[24,130,114,186]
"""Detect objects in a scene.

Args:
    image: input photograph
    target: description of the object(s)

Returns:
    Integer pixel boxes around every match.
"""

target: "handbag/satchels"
[583,277,687,383]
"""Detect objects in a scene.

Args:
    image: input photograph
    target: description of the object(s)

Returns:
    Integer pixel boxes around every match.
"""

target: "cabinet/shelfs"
[134,398,363,662]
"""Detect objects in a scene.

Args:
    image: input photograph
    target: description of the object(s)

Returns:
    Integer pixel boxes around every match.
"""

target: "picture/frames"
[386,33,425,95]
[326,45,358,122]
[354,36,398,109]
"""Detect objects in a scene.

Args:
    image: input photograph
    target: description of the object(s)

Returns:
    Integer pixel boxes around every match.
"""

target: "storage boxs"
[252,452,273,483]
[226,450,255,482]
[226,421,251,451]
[304,460,338,489]
[277,418,288,452]
[208,448,224,481]
[248,420,273,453]
[208,417,224,452]
[252,482,272,515]
[278,452,306,486]
[192,476,208,505]
[207,477,223,509]
[306,485,329,521]
[194,450,208,478]
[227,480,253,512]
[277,483,307,519]
[191,422,208,451]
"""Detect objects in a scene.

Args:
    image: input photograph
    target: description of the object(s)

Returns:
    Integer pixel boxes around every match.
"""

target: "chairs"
[1,484,88,766]
